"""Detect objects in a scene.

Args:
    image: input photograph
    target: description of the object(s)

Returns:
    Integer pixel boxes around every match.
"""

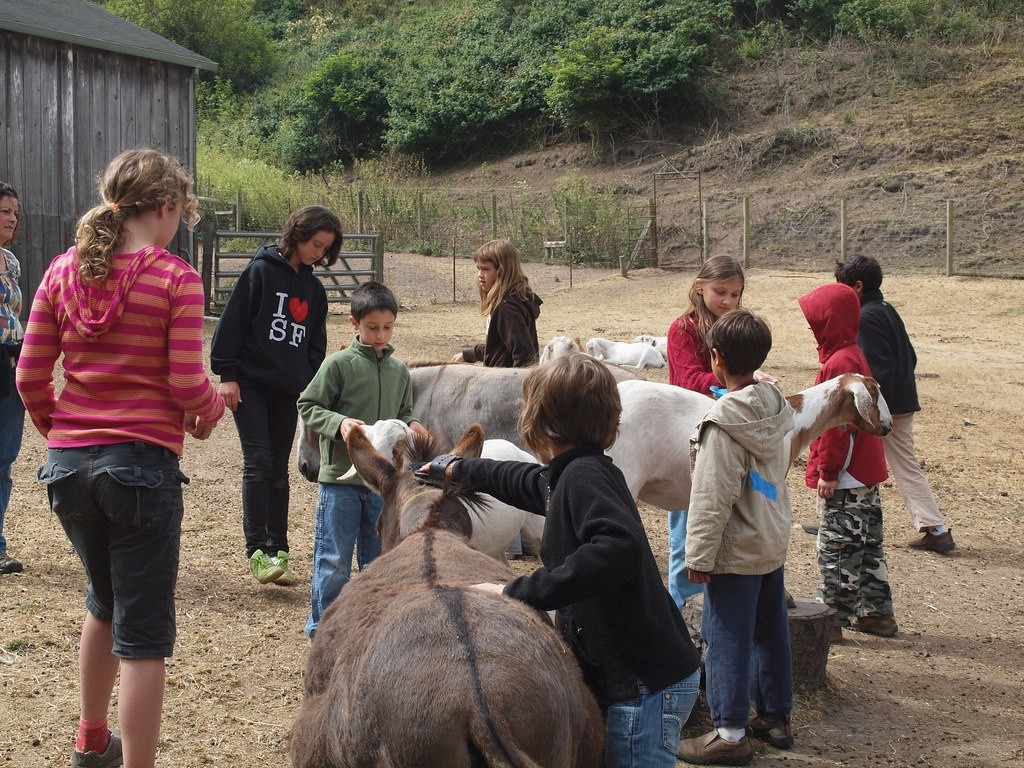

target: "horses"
[296,342,549,484]
[291,422,608,766]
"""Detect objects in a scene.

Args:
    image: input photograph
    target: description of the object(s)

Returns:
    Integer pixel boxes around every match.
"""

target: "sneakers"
[745,715,793,747]
[676,728,753,765]
[272,550,294,585]
[249,549,284,585]
[70,730,124,768]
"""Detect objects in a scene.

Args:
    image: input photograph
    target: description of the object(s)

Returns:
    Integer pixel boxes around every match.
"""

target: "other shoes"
[909,527,956,551]
[0,553,23,573]
[843,614,899,637]
[802,522,819,534]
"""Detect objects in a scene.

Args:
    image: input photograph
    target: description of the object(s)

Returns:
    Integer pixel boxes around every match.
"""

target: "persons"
[676,307,794,762]
[209,200,344,587]
[800,252,955,555]
[15,147,238,768]
[666,255,777,610]
[420,352,702,768]
[798,284,901,637]
[298,281,434,647]
[447,239,544,561]
[0,181,25,573]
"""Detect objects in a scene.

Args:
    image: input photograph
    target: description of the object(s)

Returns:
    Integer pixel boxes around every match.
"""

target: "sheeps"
[336,417,550,569]
[587,336,666,371]
[539,336,585,368]
[606,374,893,611]
